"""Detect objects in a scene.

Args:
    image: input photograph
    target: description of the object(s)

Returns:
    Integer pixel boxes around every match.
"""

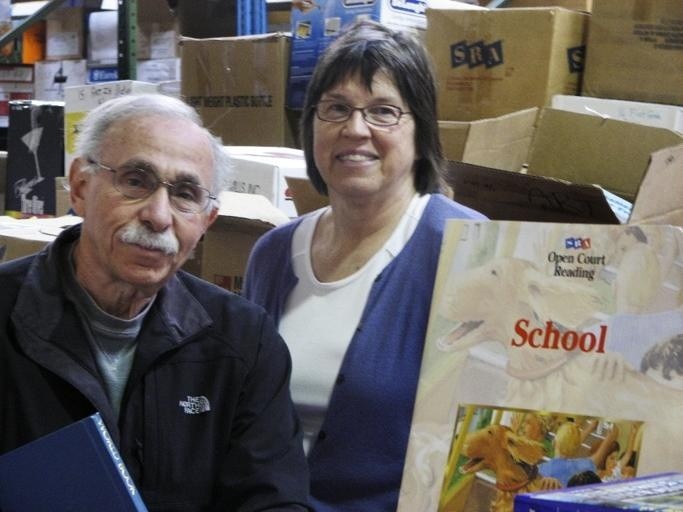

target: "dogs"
[435,258,683,475]
[460,424,566,512]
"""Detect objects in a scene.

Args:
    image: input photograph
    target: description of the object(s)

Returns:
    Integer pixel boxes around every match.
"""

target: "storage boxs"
[66,80,160,166]
[90,68,125,81]
[177,34,301,153]
[182,190,287,298]
[54,174,74,216]
[0,67,36,122]
[629,145,683,228]
[7,99,66,217]
[529,95,683,225]
[46,9,88,60]
[0,19,45,67]
[425,3,586,121]
[286,173,334,213]
[35,61,89,101]
[86,11,120,66]
[1,214,84,261]
[286,0,427,111]
[207,143,313,222]
[579,0,683,104]
[436,106,537,178]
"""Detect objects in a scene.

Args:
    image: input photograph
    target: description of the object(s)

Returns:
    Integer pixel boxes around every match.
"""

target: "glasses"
[87,159,217,214]
[311,100,411,126]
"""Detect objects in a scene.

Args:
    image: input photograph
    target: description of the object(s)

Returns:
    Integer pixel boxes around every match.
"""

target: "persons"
[0,90,312,512]
[242,26,499,512]
